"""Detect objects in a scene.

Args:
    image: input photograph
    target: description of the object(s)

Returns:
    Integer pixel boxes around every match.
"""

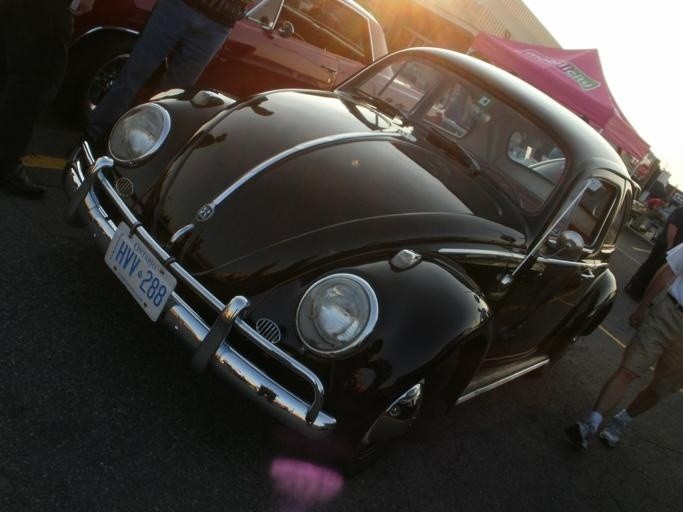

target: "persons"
[0,1,94,200]
[626,203,682,303]
[88,2,245,143]
[561,241,681,450]
[646,198,671,230]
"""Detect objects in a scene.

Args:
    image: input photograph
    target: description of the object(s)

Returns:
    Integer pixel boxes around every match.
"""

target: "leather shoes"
[0,158,48,200]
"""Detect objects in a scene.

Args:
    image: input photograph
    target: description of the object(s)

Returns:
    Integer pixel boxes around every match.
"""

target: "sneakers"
[598,413,632,452]
[625,283,654,308]
[565,420,598,450]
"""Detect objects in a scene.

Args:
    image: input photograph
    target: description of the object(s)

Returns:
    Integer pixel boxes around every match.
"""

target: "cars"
[61,43,638,482]
[40,1,444,136]
